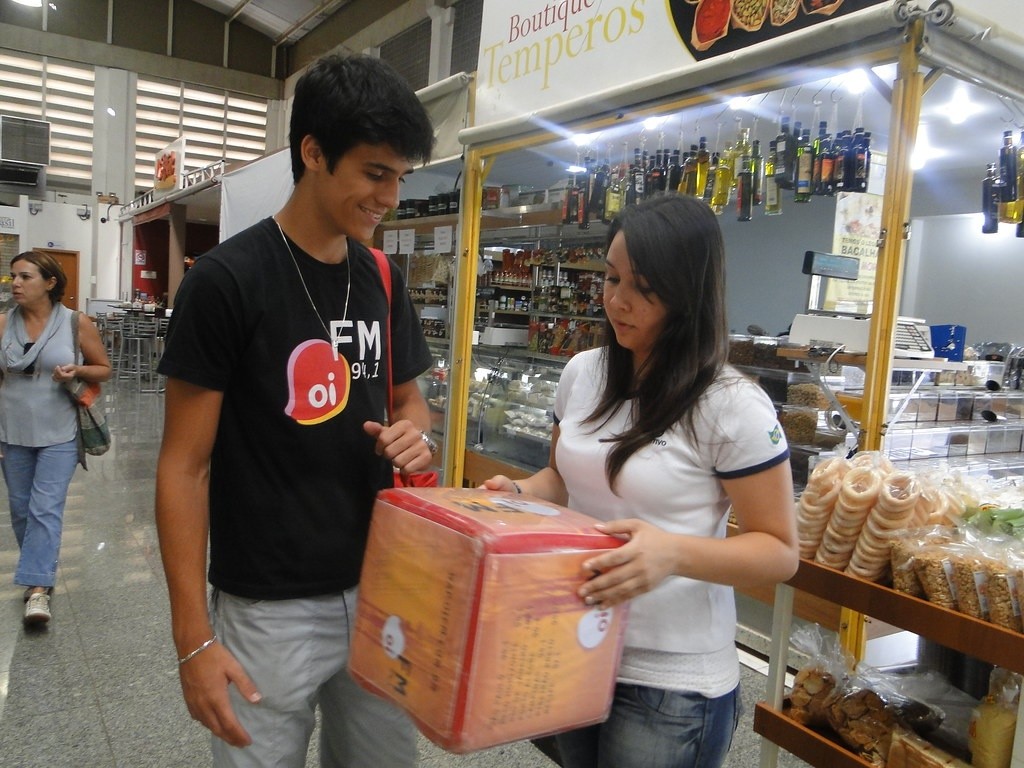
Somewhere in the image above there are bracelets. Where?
[178,635,217,663]
[511,482,522,493]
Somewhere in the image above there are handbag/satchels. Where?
[63,310,111,456]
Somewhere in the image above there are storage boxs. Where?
[348,488,631,756]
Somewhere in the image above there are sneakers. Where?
[24,592,51,624]
[22,586,37,602]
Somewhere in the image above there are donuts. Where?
[796,452,949,583]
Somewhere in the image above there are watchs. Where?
[417,429,438,456]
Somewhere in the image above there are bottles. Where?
[559,94,871,230]
[476,266,614,354]
[981,123,1023,238]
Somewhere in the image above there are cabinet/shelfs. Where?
[727,333,1024,501]
[480,270,529,348]
[752,557,1024,768]
[416,336,574,473]
[529,257,606,357]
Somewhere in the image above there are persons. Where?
[153,57,435,766]
[0,251,112,623]
[483,194,800,767]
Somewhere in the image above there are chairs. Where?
[96,302,171,394]
